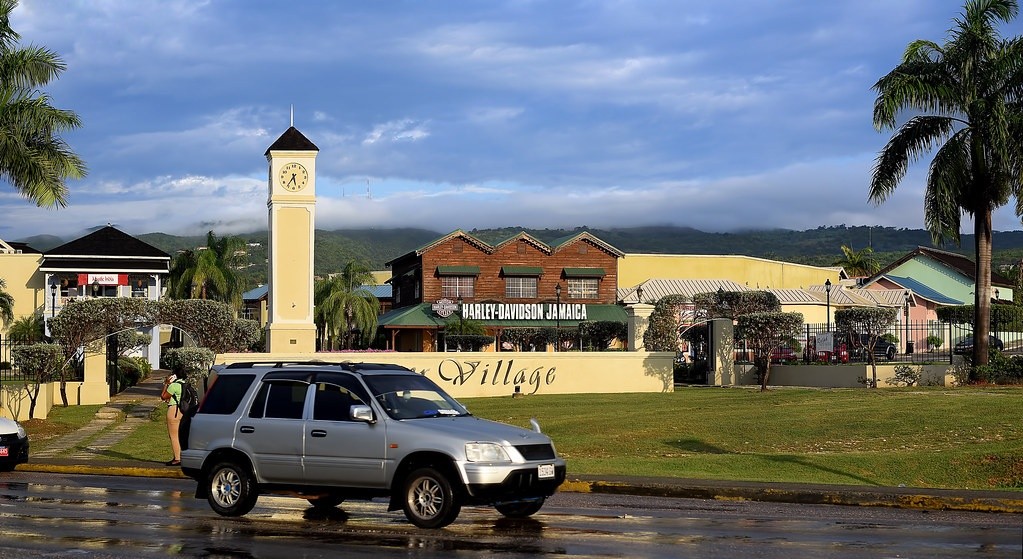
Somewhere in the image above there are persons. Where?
[161,366,187,466]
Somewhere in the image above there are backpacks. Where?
[172,381,198,417]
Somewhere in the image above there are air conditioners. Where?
[10,249,22,254]
[0,249,8,254]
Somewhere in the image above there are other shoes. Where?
[166,460,181,466]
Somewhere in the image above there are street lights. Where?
[457,294,464,346]
[903,290,911,357]
[993,286,1000,351]
[553,282,562,354]
[92,278,100,299]
[636,285,644,305]
[51,282,58,321]
[824,279,833,367]
[717,286,726,316]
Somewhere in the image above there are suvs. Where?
[843,333,897,359]
[179,360,569,531]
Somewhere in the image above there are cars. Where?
[0,415,30,473]
[803,336,849,364]
[767,341,798,363]
[674,347,687,377]
[954,333,1004,356]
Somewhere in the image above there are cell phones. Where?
[168,374,177,383]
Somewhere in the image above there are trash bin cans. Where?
[907,343,914,354]
[161,342,183,367]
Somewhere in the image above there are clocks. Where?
[279,162,308,192]
